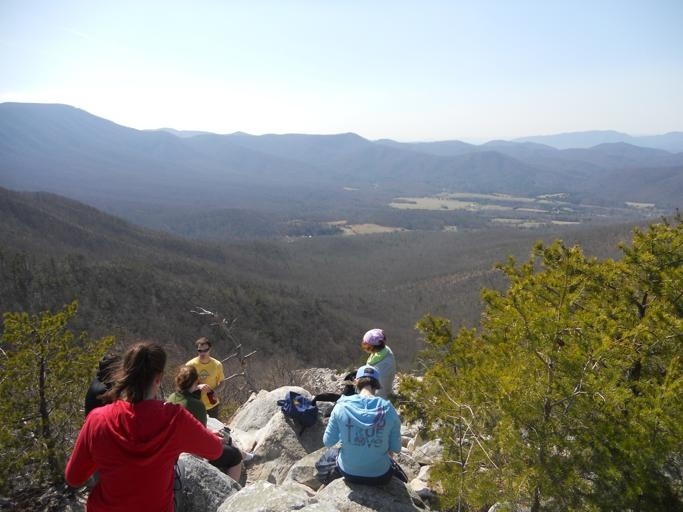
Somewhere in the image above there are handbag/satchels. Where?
[277,390,319,425]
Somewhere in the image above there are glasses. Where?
[197,347,210,353]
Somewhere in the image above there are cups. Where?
[208,390,218,405]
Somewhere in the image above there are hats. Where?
[362,328,385,346]
[355,365,380,382]
[98,352,121,369]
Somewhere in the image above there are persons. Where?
[61,341,223,511]
[358,327,397,395]
[309,363,408,485]
[165,362,206,431]
[176,335,223,420]
[201,429,244,489]
[82,351,122,420]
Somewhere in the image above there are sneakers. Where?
[386,456,407,484]
